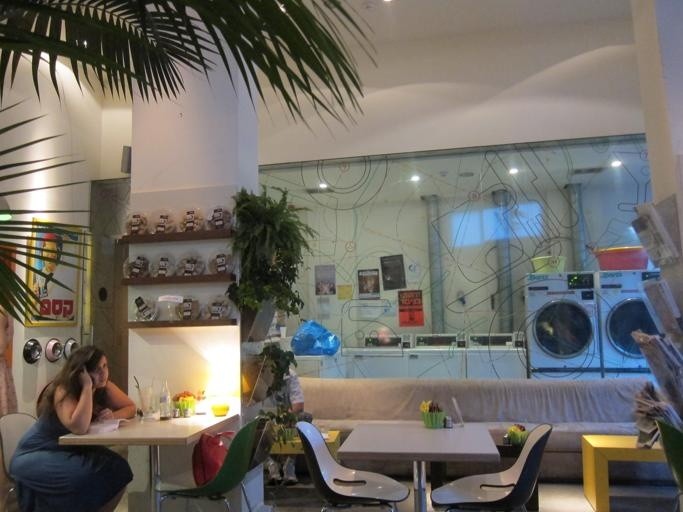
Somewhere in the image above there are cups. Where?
[279,326,286,338]
[171,408,179,418]
[182,408,190,418]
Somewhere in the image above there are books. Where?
[616,193,683,450]
[87,417,132,434]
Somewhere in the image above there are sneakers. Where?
[268,461,297,486]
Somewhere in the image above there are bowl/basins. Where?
[530,256,567,274]
[209,403,228,416]
[592,247,649,271]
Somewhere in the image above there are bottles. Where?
[159,379,170,421]
[122,205,236,318]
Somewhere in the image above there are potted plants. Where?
[233,184,320,342]
[241,347,298,448]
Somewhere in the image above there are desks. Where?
[271,430,341,466]
[582,435,669,512]
[57,411,239,512]
[337,425,500,512]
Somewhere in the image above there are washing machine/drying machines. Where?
[595,268,664,377]
[525,270,601,380]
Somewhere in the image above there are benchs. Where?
[295,379,676,484]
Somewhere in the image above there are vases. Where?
[177,402,195,416]
[423,413,444,427]
[284,429,296,439]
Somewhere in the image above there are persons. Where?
[9,345,137,510]
[257,358,304,487]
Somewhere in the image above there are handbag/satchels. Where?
[192,432,232,486]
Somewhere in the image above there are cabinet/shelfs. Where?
[120,229,237,328]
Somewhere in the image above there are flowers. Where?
[418,399,443,412]
[173,390,196,402]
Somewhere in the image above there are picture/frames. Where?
[26,218,83,326]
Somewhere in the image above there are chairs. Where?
[0,414,41,512]
[295,421,410,512]
[431,424,553,512]
[156,416,263,512]
